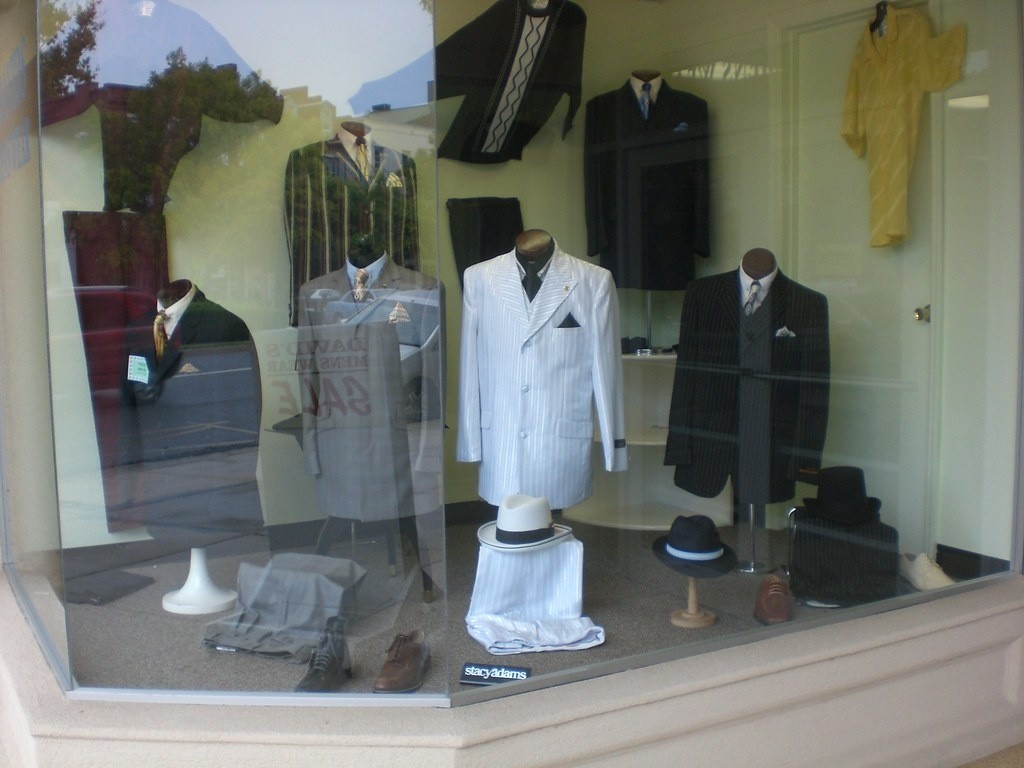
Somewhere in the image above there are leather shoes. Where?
[754,574,791,625]
[901,552,958,591]
[372,630,432,694]
[294,615,353,692]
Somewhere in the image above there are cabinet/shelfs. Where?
[560,352,735,532]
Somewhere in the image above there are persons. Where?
[118,278,266,543]
[664,247,830,503]
[297,232,448,521]
[582,65,712,292]
[460,227,636,514]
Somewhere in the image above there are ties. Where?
[516,245,554,303]
[353,269,369,301]
[641,83,653,120]
[153,311,168,362]
[743,281,761,316]
[354,135,372,182]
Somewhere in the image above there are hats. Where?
[652,514,737,578]
[802,465,882,526]
[475,493,573,553]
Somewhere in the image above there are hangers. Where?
[869,0,887,34]
